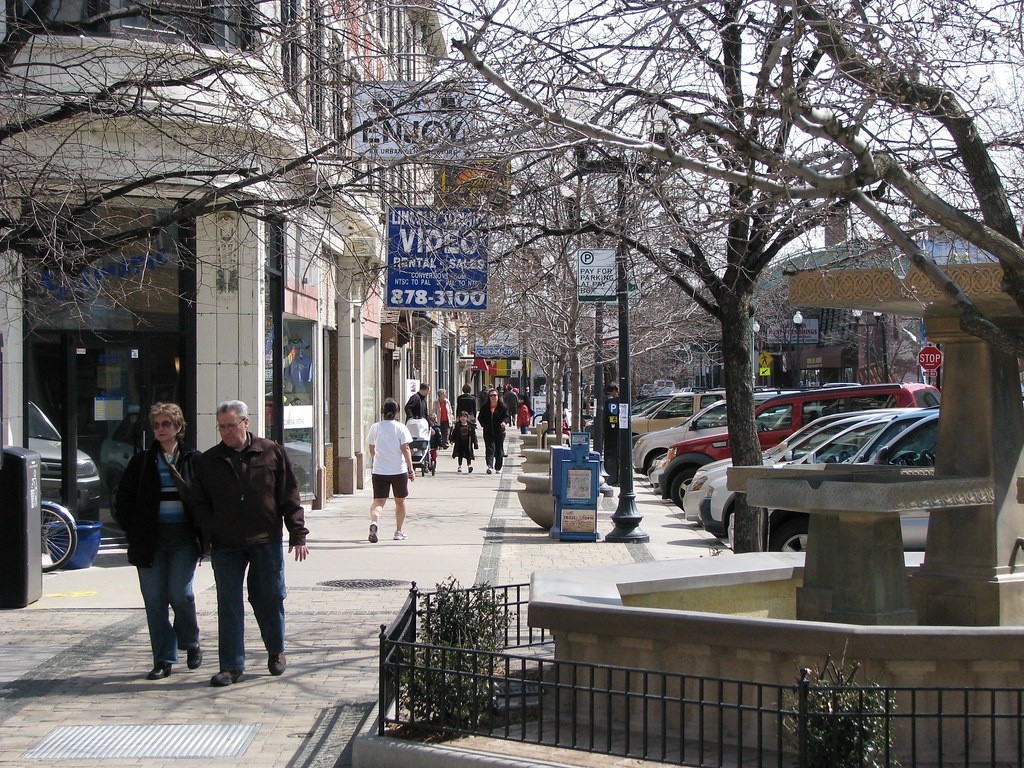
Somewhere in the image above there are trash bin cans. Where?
[0,445,43,609]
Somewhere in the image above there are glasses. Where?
[152,420,174,430]
[215,419,246,433]
[489,394,498,397]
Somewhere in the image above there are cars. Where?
[98,406,150,525]
[631,380,946,556]
[27,399,102,552]
[280,436,314,492]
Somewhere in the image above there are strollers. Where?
[404,417,438,479]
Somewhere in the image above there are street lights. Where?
[848,309,882,385]
[793,311,803,388]
[582,158,652,544]
[750,315,762,389]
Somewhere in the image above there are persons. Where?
[428,413,443,460]
[477,389,509,474]
[114,402,202,680]
[450,411,479,473]
[610,386,619,398]
[368,399,416,543]
[404,383,435,473]
[433,389,453,450]
[563,415,570,447]
[456,384,476,421]
[477,384,530,434]
[192,400,309,686]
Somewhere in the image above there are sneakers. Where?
[210,668,246,685]
[267,649,287,676]
[368,521,379,543]
[393,531,408,540]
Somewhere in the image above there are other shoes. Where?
[487,467,493,474]
[496,470,500,473]
[509,423,511,427]
[513,421,515,426]
[458,467,462,472]
[468,466,473,473]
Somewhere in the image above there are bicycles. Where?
[37,499,80,575]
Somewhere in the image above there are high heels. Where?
[187,640,202,669]
[147,662,172,680]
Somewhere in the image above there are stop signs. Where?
[917,346,944,370]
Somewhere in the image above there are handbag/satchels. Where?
[529,408,535,416]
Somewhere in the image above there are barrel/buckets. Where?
[43,519,103,570]
